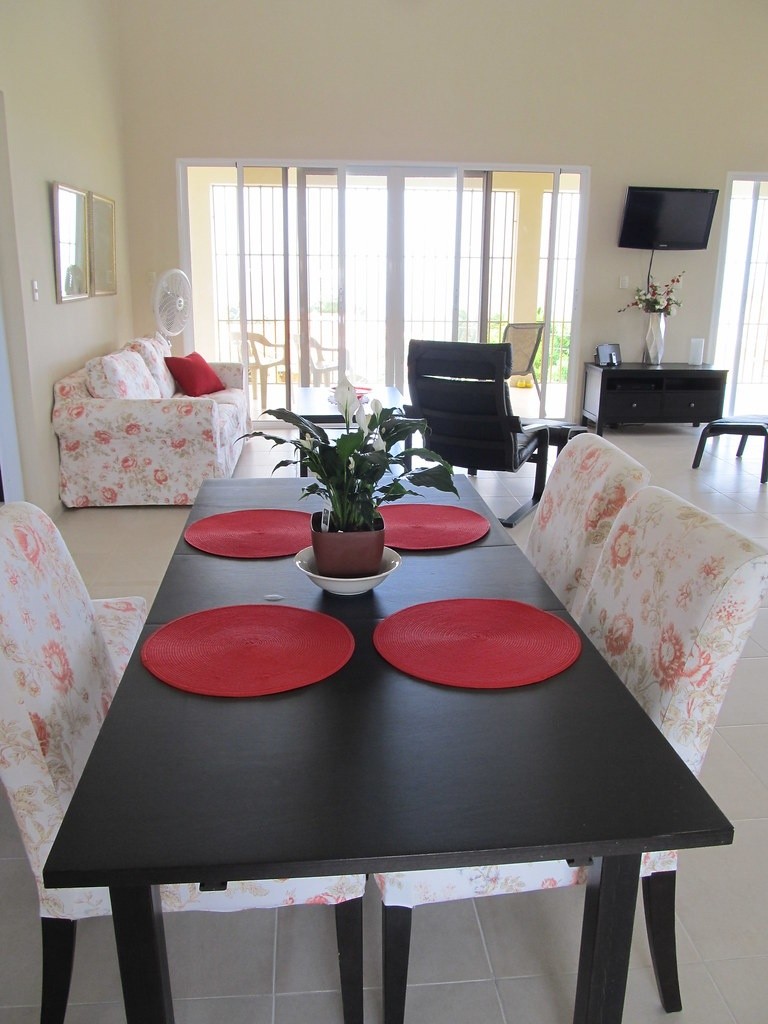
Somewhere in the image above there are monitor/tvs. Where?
[618,186,720,250]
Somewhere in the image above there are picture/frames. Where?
[88,189,117,297]
[51,180,90,304]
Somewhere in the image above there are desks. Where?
[42,471,733,1024]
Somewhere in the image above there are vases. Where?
[294,510,406,596]
[645,312,666,365]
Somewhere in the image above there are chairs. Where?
[525,432,652,623]
[229,331,296,411]
[0,502,370,1024]
[373,480,768,1024]
[501,322,547,417]
[91,591,149,675]
[691,414,768,484]
[303,331,351,388]
[400,337,550,530]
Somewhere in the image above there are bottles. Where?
[689,338,705,365]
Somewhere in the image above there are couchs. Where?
[51,332,250,509]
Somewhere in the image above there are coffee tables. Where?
[293,385,414,477]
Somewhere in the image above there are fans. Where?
[150,267,194,338]
[63,265,84,295]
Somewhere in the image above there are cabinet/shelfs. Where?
[583,362,729,439]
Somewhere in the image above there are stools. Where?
[521,415,589,460]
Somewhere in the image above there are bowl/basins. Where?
[294,545,403,595]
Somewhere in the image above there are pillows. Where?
[164,350,226,397]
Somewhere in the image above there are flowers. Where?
[617,269,687,318]
[232,372,460,530]
